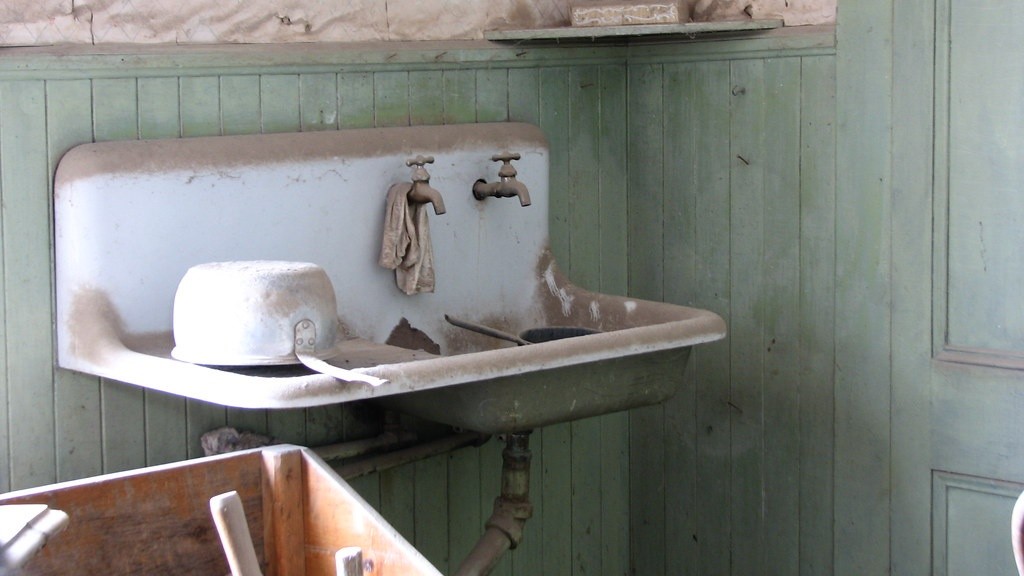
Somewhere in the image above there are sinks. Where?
[57,280,728,436]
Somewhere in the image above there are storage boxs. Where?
[0,443,440,576]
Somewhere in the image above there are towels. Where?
[376,181,436,296]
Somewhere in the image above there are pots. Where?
[171,261,390,387]
[445,312,604,346]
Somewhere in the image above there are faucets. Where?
[405,154,447,216]
[472,150,532,207]
